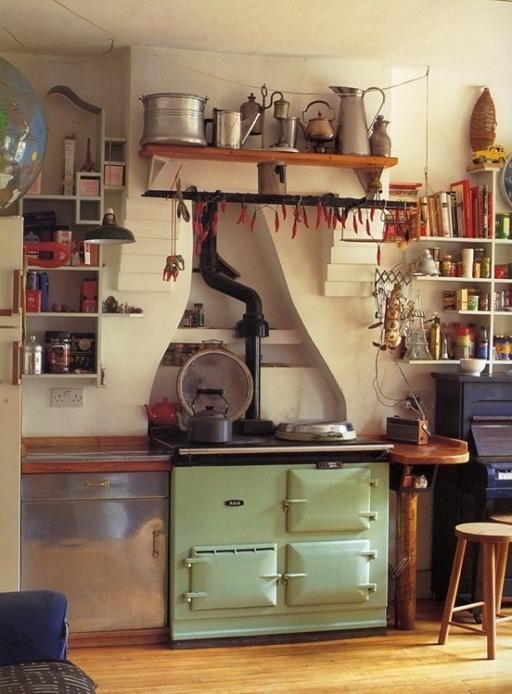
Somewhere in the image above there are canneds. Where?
[494,334,512,360]
[442,255,462,277]
[27,271,38,289]
[468,289,479,311]
[45,330,71,374]
[474,248,485,263]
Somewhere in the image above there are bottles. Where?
[194,302,204,326]
[494,334,512,361]
[467,86,496,152]
[414,249,440,274]
[441,255,452,276]
[184,308,195,328]
[483,256,491,277]
[21,225,98,373]
[426,317,488,360]
[240,91,261,134]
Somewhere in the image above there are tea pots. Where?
[144,396,183,427]
[175,387,234,446]
[328,84,385,155]
[204,106,260,149]
[370,115,391,156]
[298,98,337,153]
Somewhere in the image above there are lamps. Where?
[375,247,441,287]
[82,38,135,247]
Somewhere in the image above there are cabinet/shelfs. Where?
[19,83,145,390]
[409,164,512,377]
[21,470,169,652]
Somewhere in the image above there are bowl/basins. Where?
[458,357,486,377]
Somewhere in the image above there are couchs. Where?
[1,588,99,694]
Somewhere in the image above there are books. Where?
[421,179,495,240]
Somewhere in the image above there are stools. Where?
[491,510,509,614]
[435,521,510,661]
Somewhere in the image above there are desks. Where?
[358,433,473,630]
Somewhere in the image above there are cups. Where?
[467,290,511,311]
[451,247,480,277]
[257,158,287,195]
[279,116,299,147]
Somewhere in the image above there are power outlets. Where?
[50,386,83,408]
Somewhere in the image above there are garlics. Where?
[386,289,402,348]
